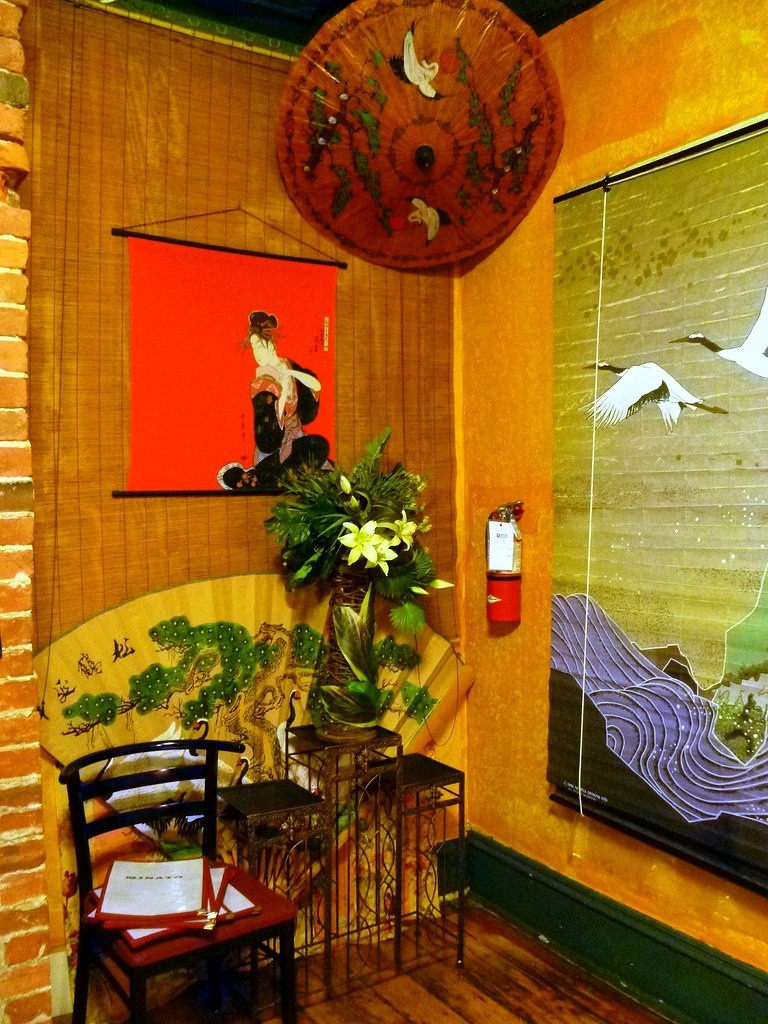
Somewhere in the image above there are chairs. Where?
[59,738,298,1024]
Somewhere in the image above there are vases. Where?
[306,585,382,743]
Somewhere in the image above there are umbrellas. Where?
[275,0,566,268]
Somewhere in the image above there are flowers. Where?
[261,434,454,599]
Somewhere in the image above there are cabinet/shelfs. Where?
[218,725,465,1010]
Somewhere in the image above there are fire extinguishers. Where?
[485,499,525,624]
